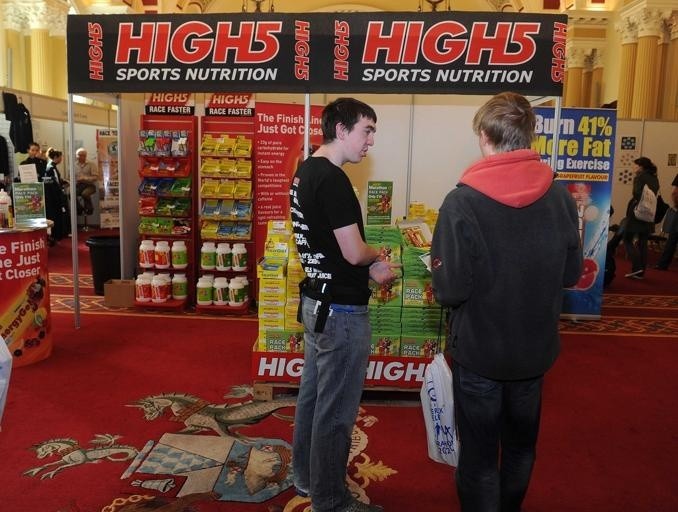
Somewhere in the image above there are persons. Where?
[0,135,98,241]
[624,157,660,280]
[289,97,401,512]
[432,93,583,511]
[652,173,678,270]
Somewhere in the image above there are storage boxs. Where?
[103,278,137,307]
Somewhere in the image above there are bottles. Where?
[133,272,190,304]
[138,239,189,270]
[200,241,251,272]
[194,274,249,307]
[0,188,13,229]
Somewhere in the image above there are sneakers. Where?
[624,267,644,281]
[335,489,380,512]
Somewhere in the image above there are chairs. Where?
[647,209,676,258]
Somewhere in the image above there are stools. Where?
[75,193,95,234]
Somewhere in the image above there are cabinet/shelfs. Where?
[134,112,198,315]
[199,115,254,314]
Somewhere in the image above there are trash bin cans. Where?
[86,235,121,296]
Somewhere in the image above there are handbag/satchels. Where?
[662,208,678,233]
[420,349,461,469]
[655,194,669,223]
[633,183,656,222]
